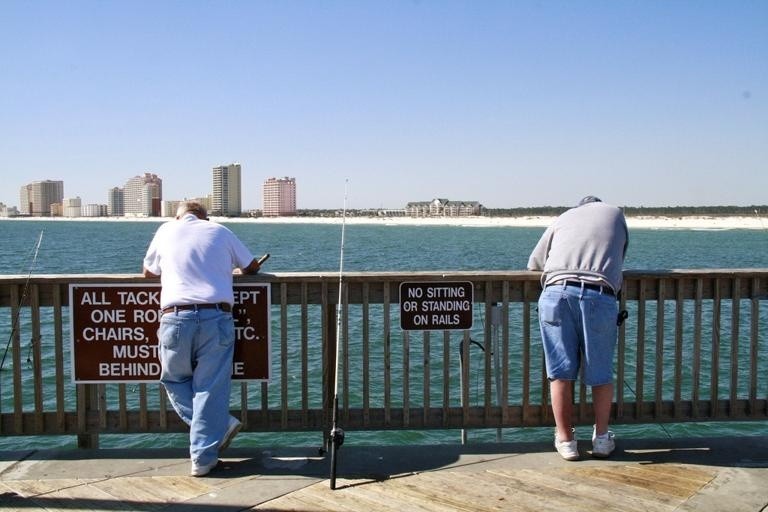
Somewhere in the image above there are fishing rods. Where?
[1,231,43,375]
[320,181,346,490]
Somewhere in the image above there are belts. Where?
[163,305,222,312]
[554,280,614,295]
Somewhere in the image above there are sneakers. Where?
[554,427,580,460]
[592,424,616,457]
[192,457,218,476]
[219,419,242,451]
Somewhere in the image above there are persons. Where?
[521,191,630,464]
[140,201,260,480]
